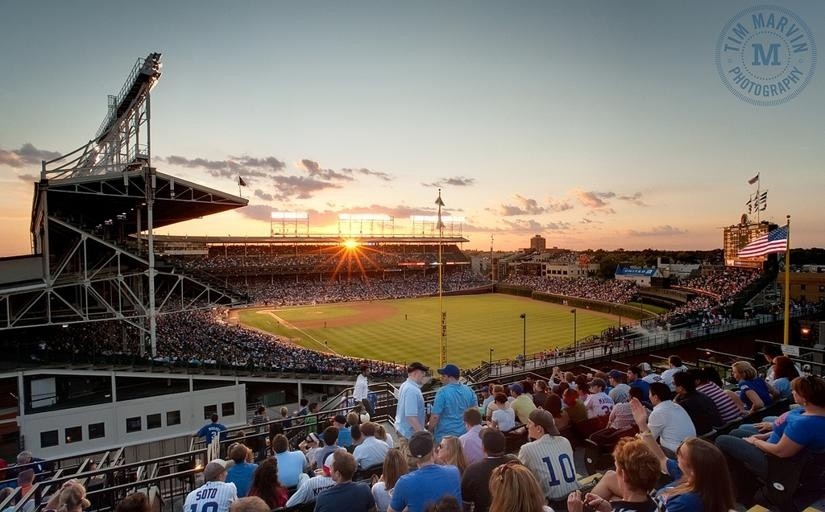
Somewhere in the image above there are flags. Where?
[748,175,759,185]
[738,224,789,258]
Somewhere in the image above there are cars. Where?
[781,263,825,274]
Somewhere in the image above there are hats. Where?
[508,384,522,393]
[479,428,506,451]
[206,459,234,480]
[438,365,459,378]
[408,363,429,373]
[638,363,652,374]
[409,430,433,458]
[529,408,560,435]
[587,371,622,385]
[331,415,346,424]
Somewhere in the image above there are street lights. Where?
[519,312,528,359]
[488,347,495,363]
[96,51,163,152]
[569,307,578,348]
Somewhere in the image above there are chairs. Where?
[270,382,825,512]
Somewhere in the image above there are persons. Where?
[539,348,559,367]
[17,286,294,370]
[659,297,757,338]
[393,361,825,512]
[679,267,763,302]
[157,244,486,304]
[773,299,817,318]
[352,367,375,418]
[505,271,642,306]
[1,450,103,512]
[117,492,149,512]
[600,326,630,354]
[290,348,407,374]
[184,399,393,512]
